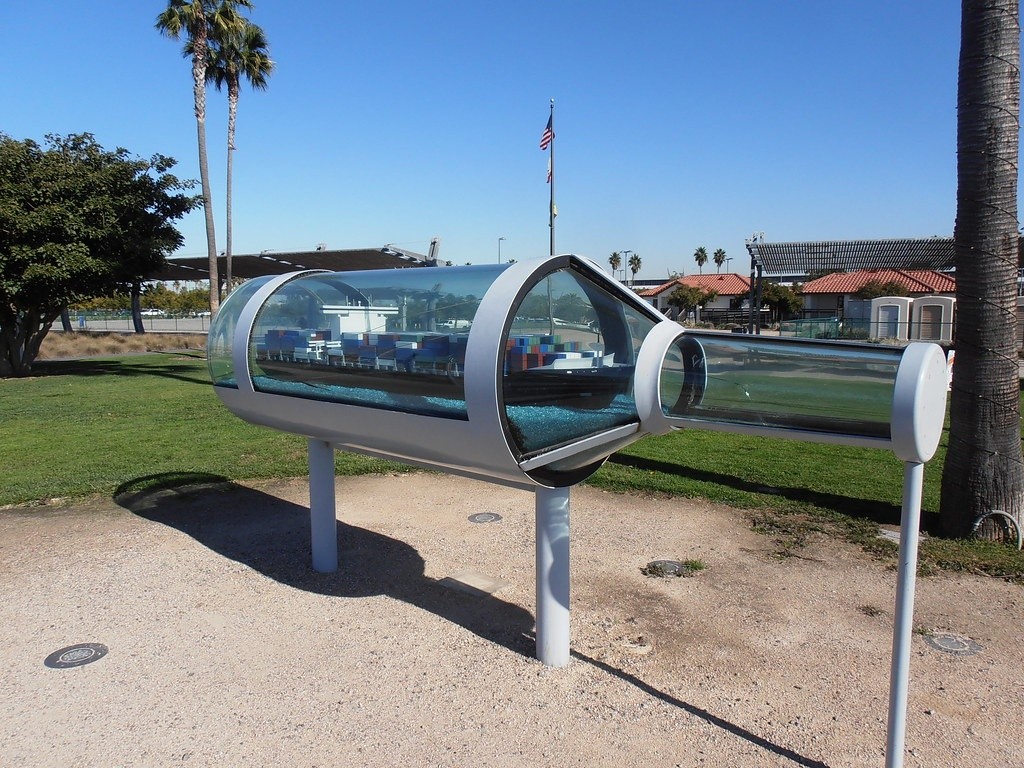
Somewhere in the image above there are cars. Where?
[189,310,211,318]
[140,308,165,316]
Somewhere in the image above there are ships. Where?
[253,303,638,410]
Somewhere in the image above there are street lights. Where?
[620,249,633,287]
[498,237,506,264]
[725,257,734,274]
[617,269,624,282]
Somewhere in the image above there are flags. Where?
[539,112,555,151]
